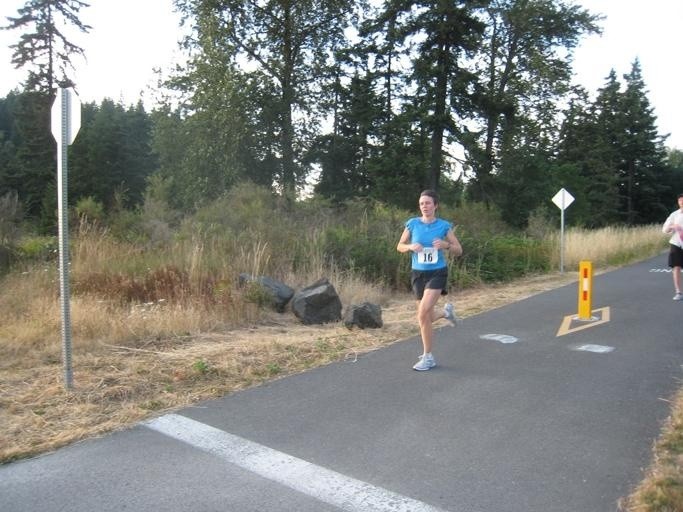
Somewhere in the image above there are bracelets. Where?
[446,240,451,250]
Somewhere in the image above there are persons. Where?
[397,190,462,371]
[662,194,683,300]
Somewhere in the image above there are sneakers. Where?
[413,354,436,371]
[673,293,683,300]
[444,304,457,327]
